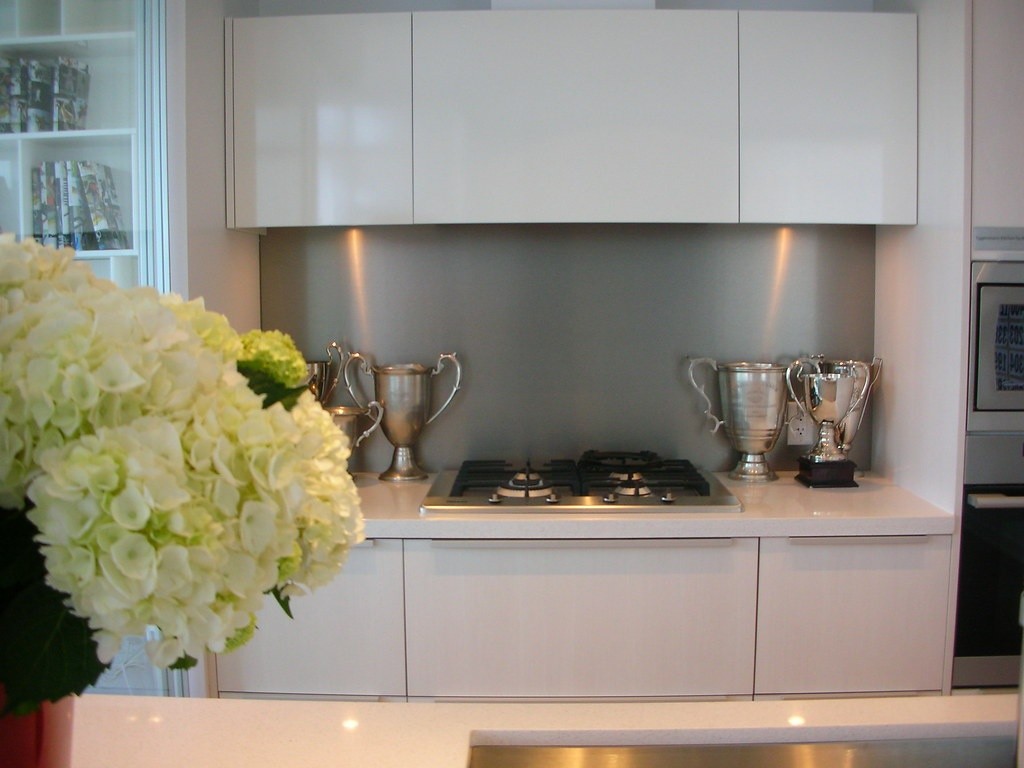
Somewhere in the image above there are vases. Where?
[0,684,75,768]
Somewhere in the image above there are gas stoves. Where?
[418,450,745,513]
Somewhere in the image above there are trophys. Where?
[324,401,384,453]
[308,343,345,409]
[689,358,805,483]
[784,354,882,489]
[345,353,462,480]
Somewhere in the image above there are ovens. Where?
[954,482,1024,691]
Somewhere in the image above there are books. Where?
[0,55,91,133]
[31,160,129,251]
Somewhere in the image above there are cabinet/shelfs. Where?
[0,0,156,289]
[208,538,407,705]
[753,535,953,701]
[401,538,758,699]
[224,10,919,236]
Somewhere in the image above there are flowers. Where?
[0,232,368,717]
